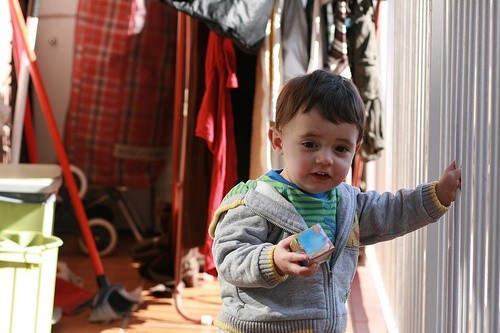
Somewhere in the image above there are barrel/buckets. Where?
[0,230,63,332]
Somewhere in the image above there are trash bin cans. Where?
[0,231,64,333]
[0,163,63,239]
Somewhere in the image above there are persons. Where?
[208,70,463,333]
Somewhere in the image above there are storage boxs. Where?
[1,163,63,238]
